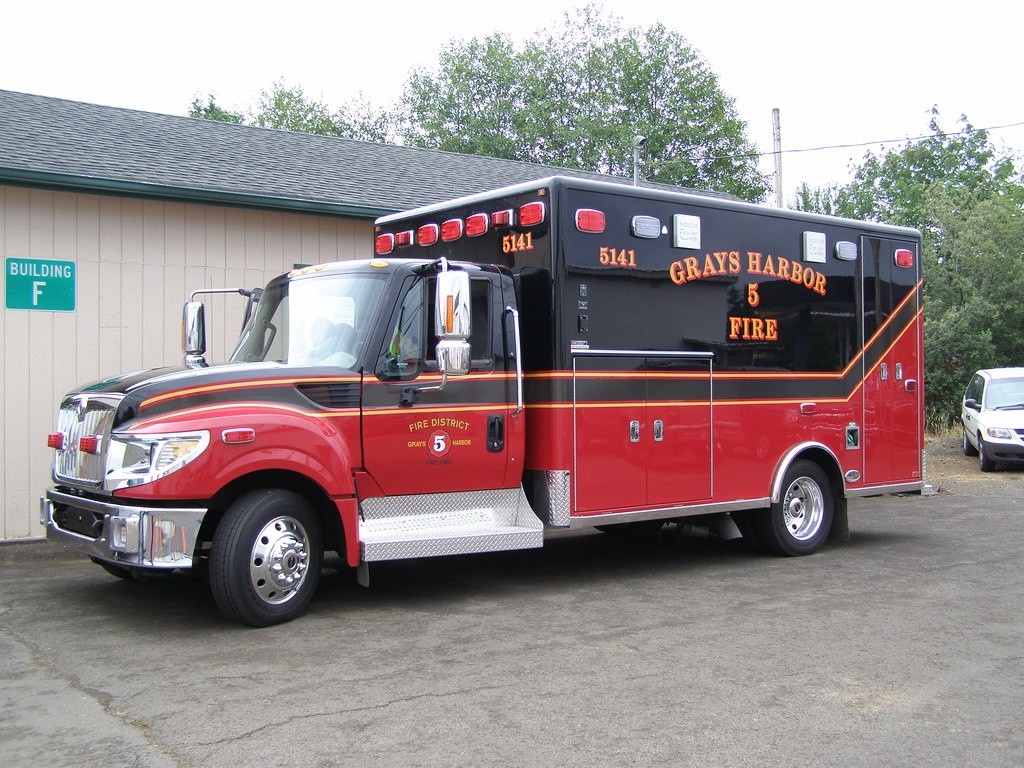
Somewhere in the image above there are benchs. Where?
[425,297,488,361]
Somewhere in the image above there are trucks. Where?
[41,176,936,628]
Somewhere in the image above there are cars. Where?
[961,367,1024,472]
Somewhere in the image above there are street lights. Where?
[633,135,646,187]
